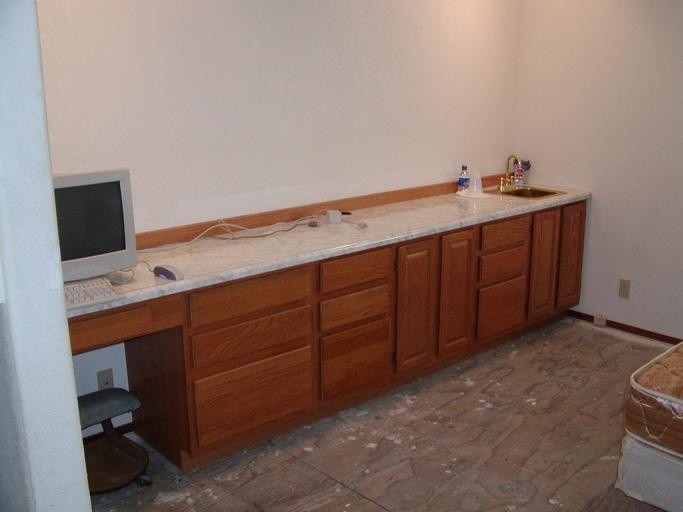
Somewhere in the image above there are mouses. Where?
[153,264,184,282]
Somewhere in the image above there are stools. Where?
[76,387,153,495]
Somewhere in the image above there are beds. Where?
[615,342,683,512]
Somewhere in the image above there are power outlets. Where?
[618,277,630,300]
[96,368,114,391]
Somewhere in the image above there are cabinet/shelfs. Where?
[129,196,588,475]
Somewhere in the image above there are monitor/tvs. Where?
[52,167,138,283]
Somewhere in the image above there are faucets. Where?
[505,154,526,187]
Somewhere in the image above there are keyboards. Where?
[63,276,122,310]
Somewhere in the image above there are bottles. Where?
[458,165,469,196]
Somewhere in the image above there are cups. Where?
[469,174,483,197]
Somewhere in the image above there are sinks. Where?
[483,187,568,200]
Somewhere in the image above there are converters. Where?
[326,209,342,224]
